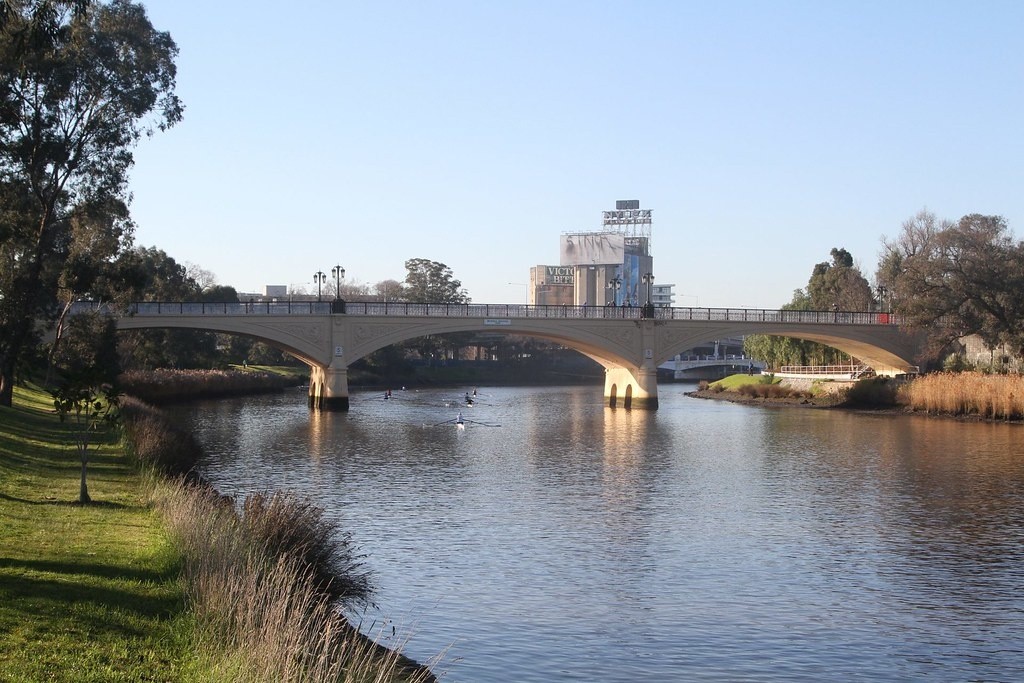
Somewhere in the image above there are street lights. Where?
[609,278,621,307]
[642,270,654,304]
[330,262,346,298]
[876,285,887,313]
[313,270,327,302]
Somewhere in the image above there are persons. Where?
[384,388,391,398]
[464,388,477,404]
[747,360,754,376]
[456,411,464,425]
[249,296,255,314]
